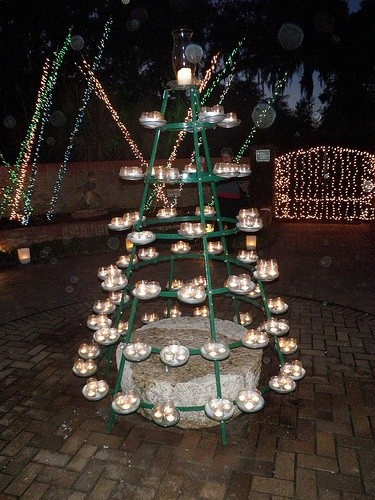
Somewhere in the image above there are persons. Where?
[204,140,277,262]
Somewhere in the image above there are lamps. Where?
[17,247,32,264]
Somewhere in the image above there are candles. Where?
[74,67,304,429]
